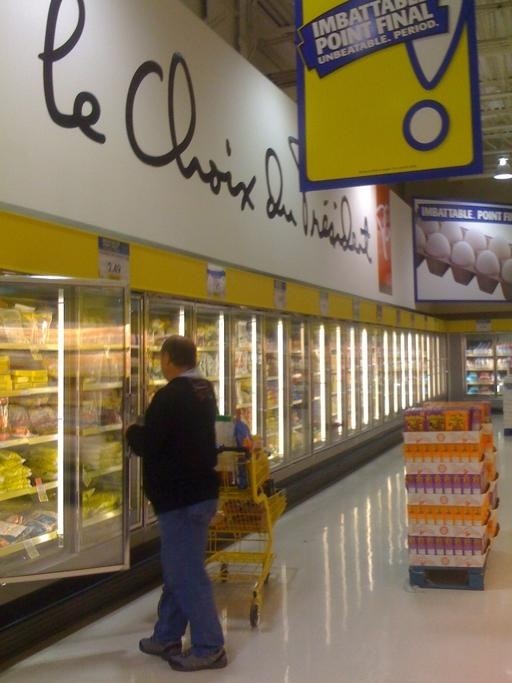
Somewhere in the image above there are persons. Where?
[123,333,229,672]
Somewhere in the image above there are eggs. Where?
[417,217,512,284]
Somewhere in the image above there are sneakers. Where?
[169,647,226,672]
[140,635,183,659]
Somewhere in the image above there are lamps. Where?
[493,155,512,180]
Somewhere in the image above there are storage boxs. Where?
[402,399,499,567]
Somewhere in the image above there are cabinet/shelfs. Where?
[465,355,512,395]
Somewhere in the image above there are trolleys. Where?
[200,445,288,627]
